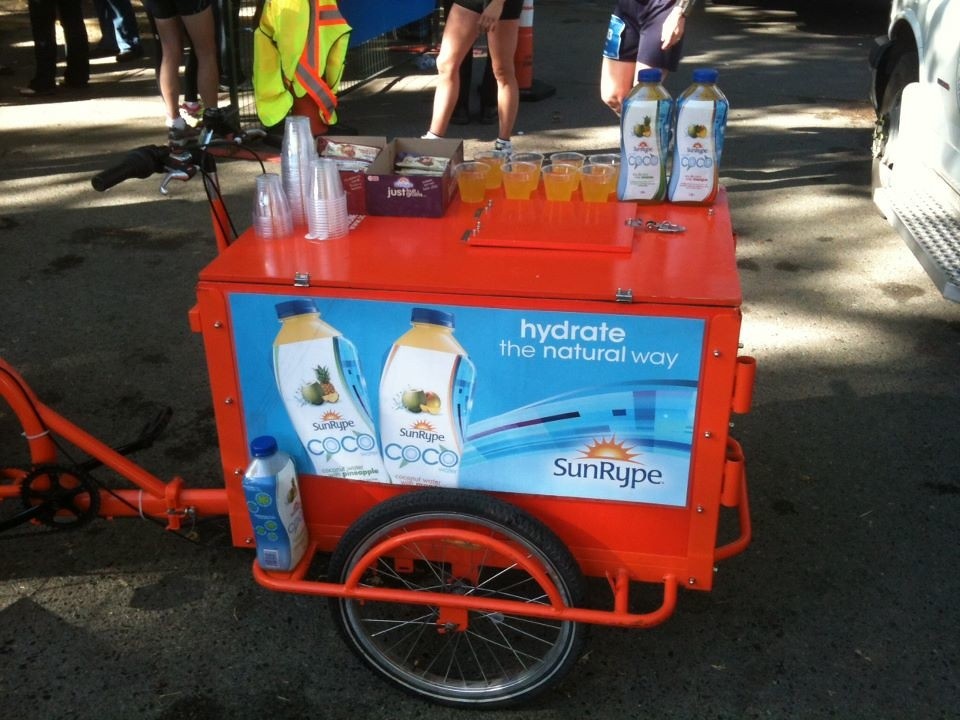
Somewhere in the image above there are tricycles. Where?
[2,108,755,705]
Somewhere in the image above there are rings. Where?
[677,38,680,40]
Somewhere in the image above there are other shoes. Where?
[492,141,512,156]
[89,47,120,58]
[56,81,88,91]
[19,78,56,96]
[116,50,142,62]
[181,103,201,116]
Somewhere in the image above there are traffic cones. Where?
[487,0,556,102]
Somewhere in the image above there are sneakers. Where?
[167,123,201,147]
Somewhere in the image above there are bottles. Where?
[615,67,674,202]
[273,299,391,483]
[667,69,729,204]
[377,308,477,487]
[393,50,435,70]
[240,436,310,572]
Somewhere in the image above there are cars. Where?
[858,1,960,312]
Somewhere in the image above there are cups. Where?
[577,164,617,202]
[540,164,579,201]
[500,162,538,200]
[509,151,544,189]
[472,150,508,188]
[550,152,586,191]
[455,162,491,203]
[304,158,350,239]
[251,173,294,239]
[588,154,620,193]
[280,115,320,225]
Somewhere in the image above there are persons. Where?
[601,0,695,118]
[421,0,523,162]
[20,0,236,145]
